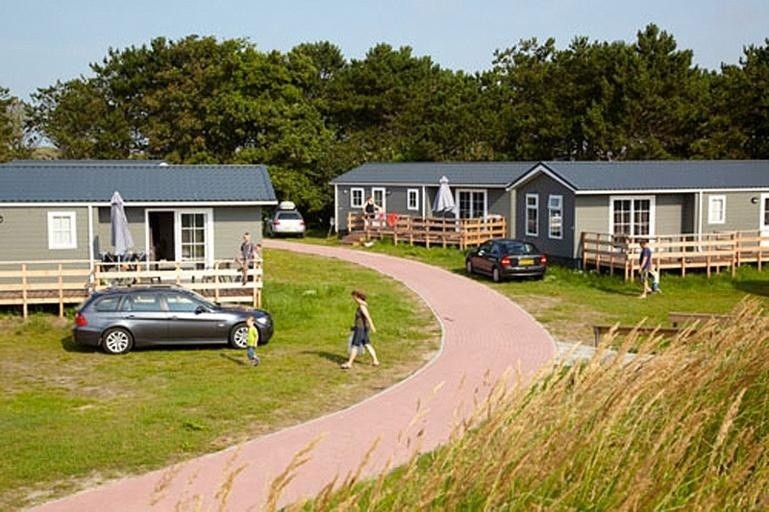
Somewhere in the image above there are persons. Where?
[363,196,375,233]
[649,265,666,295]
[341,290,381,369]
[235,232,253,285]
[254,243,263,281]
[244,314,262,367]
[373,202,386,230]
[636,240,652,298]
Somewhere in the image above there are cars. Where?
[74,283,275,354]
[466,239,547,283]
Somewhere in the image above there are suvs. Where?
[264,200,306,238]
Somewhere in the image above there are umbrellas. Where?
[110,191,134,272]
[432,176,457,218]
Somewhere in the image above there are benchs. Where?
[668,311,734,327]
[591,326,715,350]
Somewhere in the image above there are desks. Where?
[102,251,152,283]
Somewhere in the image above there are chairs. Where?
[200,257,233,293]
